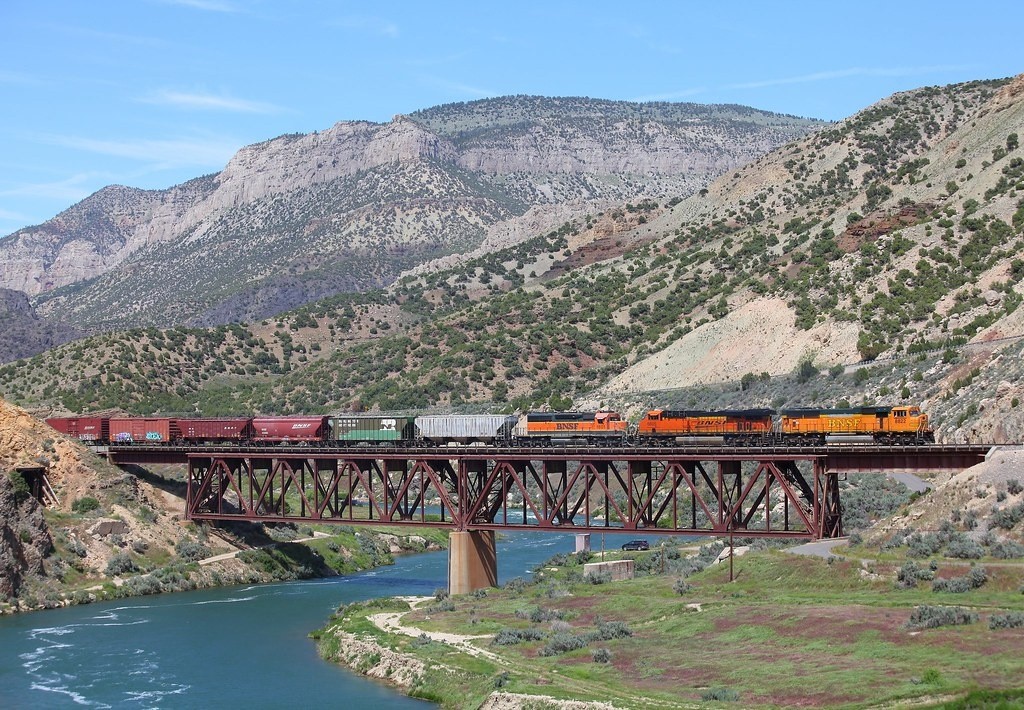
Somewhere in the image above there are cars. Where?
[339,499,362,506]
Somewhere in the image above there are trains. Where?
[46,405,936,448]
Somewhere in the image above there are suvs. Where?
[622,540,650,551]
